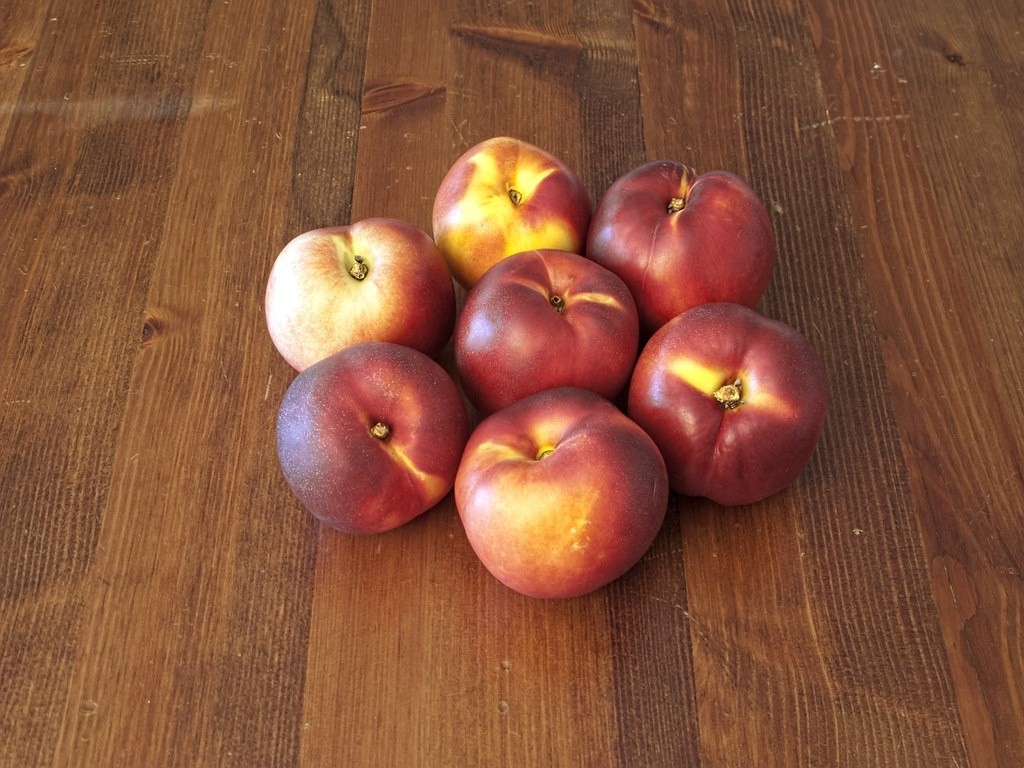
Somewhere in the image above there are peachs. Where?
[264,137,830,603]
